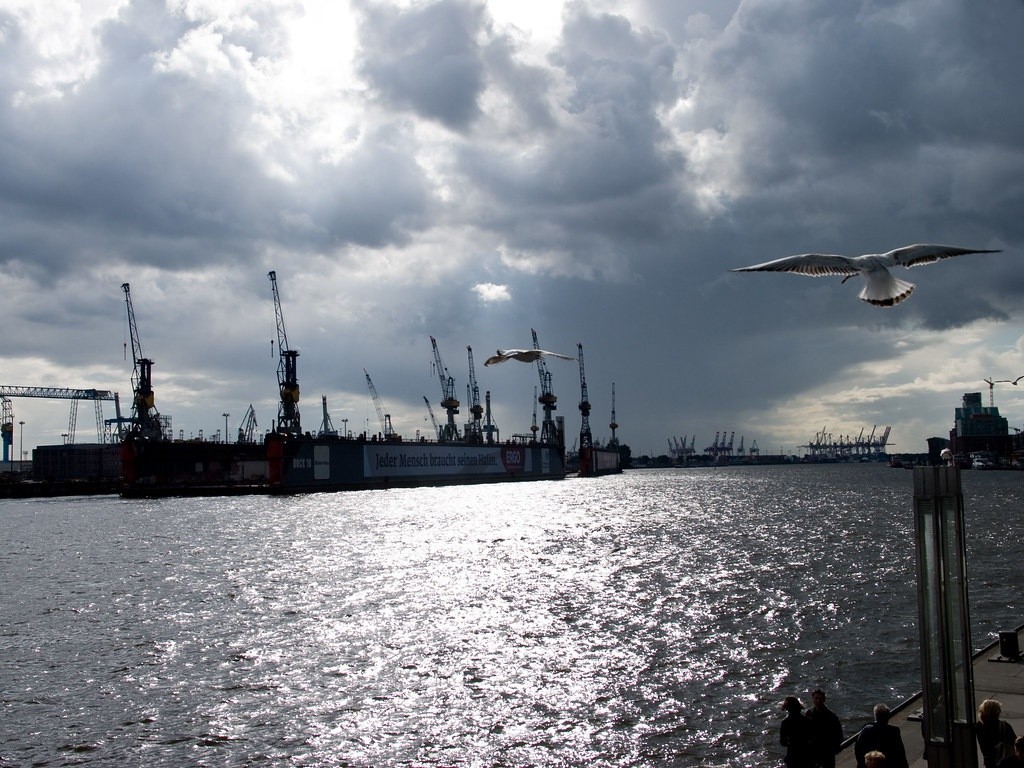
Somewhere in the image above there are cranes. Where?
[264,269,312,491]
[316,327,619,478]
[103,282,170,498]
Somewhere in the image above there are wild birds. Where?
[725,243,1007,311]
[993,375,1024,386]
[482,347,577,369]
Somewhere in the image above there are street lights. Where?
[18,421,25,473]
[222,411,231,444]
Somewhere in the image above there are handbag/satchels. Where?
[993,721,1015,766]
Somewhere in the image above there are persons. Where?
[779,688,844,768]
[854,703,909,768]
[976,698,1024,768]
